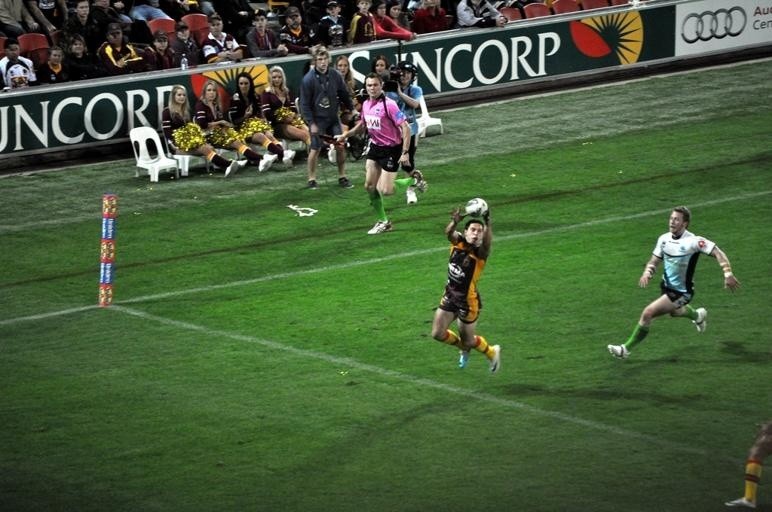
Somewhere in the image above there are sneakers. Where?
[307,179,320,190]
[692,307,707,333]
[409,170,428,195]
[723,498,758,509]
[406,188,419,205]
[257,153,279,173]
[367,218,393,235]
[224,157,248,178]
[338,177,354,190]
[282,149,297,169]
[487,344,501,372]
[607,343,632,362]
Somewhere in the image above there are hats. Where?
[251,8,266,19]
[326,0,340,8]
[106,21,124,35]
[208,13,223,22]
[174,20,190,31]
[285,7,301,18]
[151,28,170,40]
[399,60,419,74]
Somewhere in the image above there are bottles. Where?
[179,54,189,71]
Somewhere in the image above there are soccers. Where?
[465,198,489,217]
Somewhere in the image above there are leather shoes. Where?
[458,350,470,368]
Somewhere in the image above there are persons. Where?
[1,1,243,91]
[722,424,771,511]
[456,0,508,28]
[431,198,502,373]
[260,64,338,166]
[385,60,423,205]
[247,1,441,56]
[297,45,363,192]
[373,53,390,77]
[333,72,430,238]
[605,205,742,360]
[335,56,365,143]
[194,80,280,175]
[160,83,249,178]
[228,73,298,170]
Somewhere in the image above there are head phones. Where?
[398,60,418,82]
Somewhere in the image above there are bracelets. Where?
[641,270,653,280]
[723,271,733,278]
[401,150,410,156]
